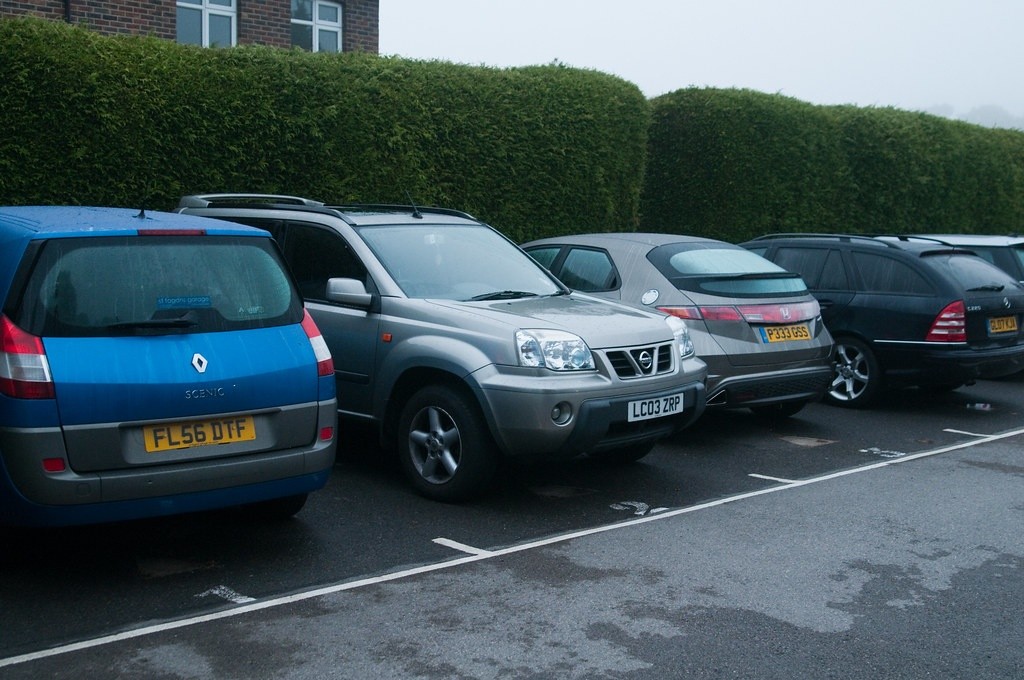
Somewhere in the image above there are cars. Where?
[0,205,338,570]
[516,232,838,433]
[874,232,1024,290]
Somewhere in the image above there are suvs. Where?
[736,232,1024,409]
[146,192,708,503]
